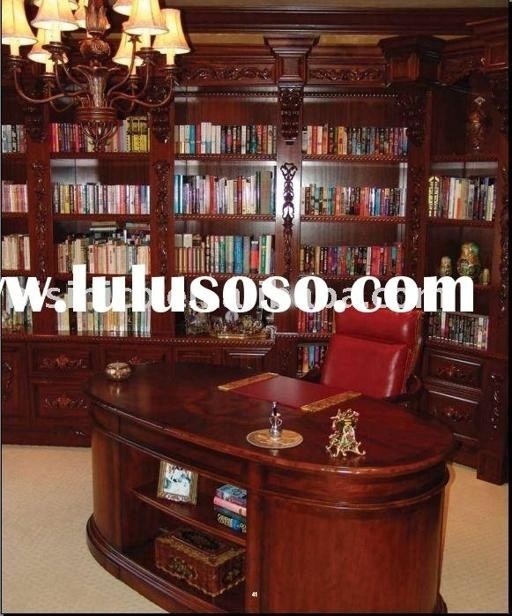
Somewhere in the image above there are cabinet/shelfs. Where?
[1,0,512,484]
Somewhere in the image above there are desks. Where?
[83,361,453,614]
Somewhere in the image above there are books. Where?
[427,307,489,352]
[0,116,408,343]
[213,483,247,534]
[295,341,328,380]
[427,172,496,221]
